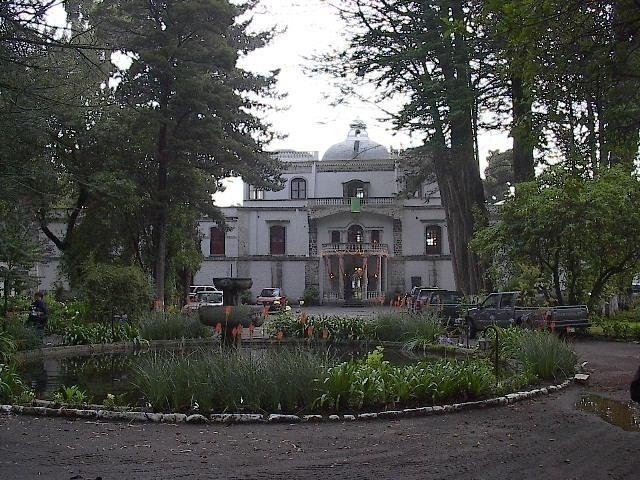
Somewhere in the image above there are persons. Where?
[28,292,48,330]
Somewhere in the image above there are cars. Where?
[183,284,224,310]
[394,285,476,326]
[256,287,288,312]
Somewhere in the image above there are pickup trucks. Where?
[458,291,592,339]
